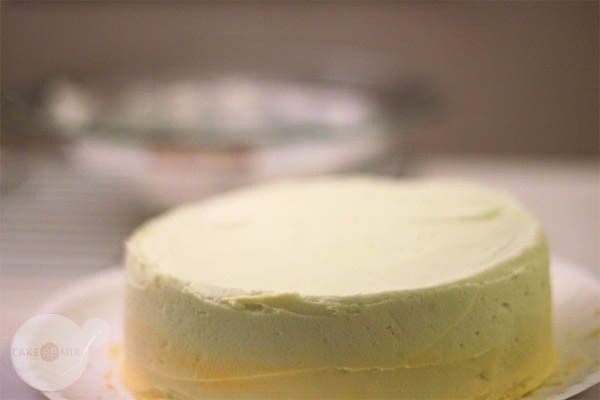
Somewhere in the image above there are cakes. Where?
[123,177,554,400]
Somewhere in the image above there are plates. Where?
[10,254,600,400]
